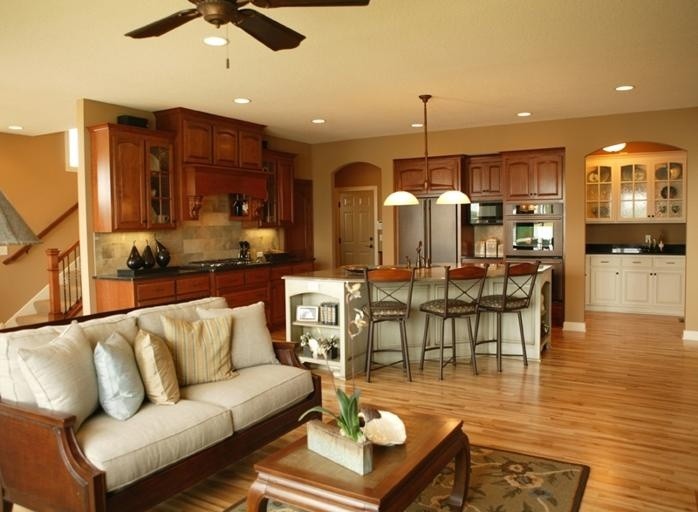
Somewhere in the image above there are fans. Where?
[122,0,370,52]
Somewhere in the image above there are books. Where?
[319,302,337,325]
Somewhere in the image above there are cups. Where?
[157,213,169,224]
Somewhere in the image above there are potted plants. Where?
[298,384,374,477]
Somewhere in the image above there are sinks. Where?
[395,265,431,270]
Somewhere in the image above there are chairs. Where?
[363,266,416,383]
[420,262,489,381]
[471,260,542,372]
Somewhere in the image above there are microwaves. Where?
[464,203,503,226]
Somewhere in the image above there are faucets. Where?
[413,240,422,268]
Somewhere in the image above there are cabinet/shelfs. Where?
[92,270,211,313]
[279,272,371,381]
[211,264,272,329]
[534,264,551,361]
[393,147,565,203]
[242,150,298,228]
[87,123,177,233]
[151,106,268,170]
[272,258,315,327]
[459,206,564,259]
[585,253,686,316]
[583,138,686,223]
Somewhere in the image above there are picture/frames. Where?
[296,304,320,323]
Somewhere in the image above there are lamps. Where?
[383,94,472,206]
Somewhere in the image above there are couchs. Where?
[0,298,322,512]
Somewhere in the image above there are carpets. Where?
[222,442,591,512]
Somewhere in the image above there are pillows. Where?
[17,300,281,433]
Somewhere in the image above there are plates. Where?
[358,408,407,448]
[632,167,646,181]
[660,184,678,198]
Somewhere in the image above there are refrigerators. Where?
[399,193,456,266]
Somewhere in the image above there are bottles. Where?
[265,198,273,222]
[233,192,249,216]
